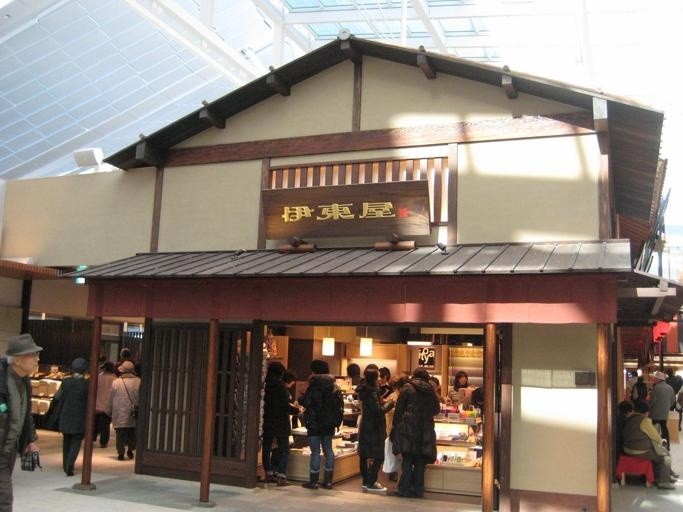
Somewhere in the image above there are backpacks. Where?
[313,378,345,431]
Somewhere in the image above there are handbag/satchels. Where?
[632,385,640,401]
[55,397,65,416]
[130,404,139,418]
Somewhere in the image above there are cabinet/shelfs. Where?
[22,365,68,434]
[286,389,360,486]
[424,413,483,497]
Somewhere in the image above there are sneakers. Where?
[670,475,677,482]
[64,443,134,476]
[263,471,275,483]
[657,481,676,490]
[362,480,369,489]
[277,474,292,487]
[366,481,388,492]
[672,470,680,476]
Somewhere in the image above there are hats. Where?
[119,348,132,358]
[4,333,44,357]
[117,361,137,374]
[309,359,329,374]
[647,370,665,381]
[412,366,429,380]
[635,401,649,413]
[71,357,89,373]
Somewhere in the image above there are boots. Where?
[302,470,319,489]
[319,468,333,489]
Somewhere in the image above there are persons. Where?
[261,358,484,497]
[45,356,90,477]
[92,347,141,461]
[619,368,682,491]
[0,332,44,512]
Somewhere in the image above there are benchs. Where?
[620,456,653,487]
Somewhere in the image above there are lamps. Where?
[359,325,373,357]
[322,326,335,357]
